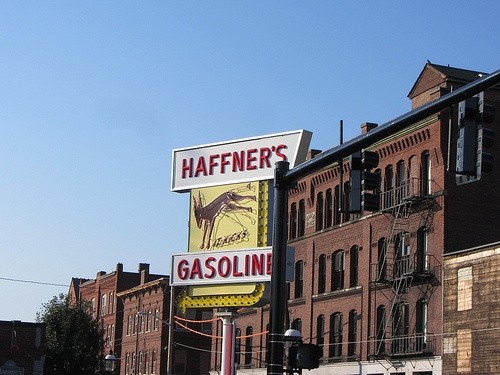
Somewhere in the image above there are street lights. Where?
[282,318,320,375]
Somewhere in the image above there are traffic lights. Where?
[455,91,484,187]
[347,150,381,214]
